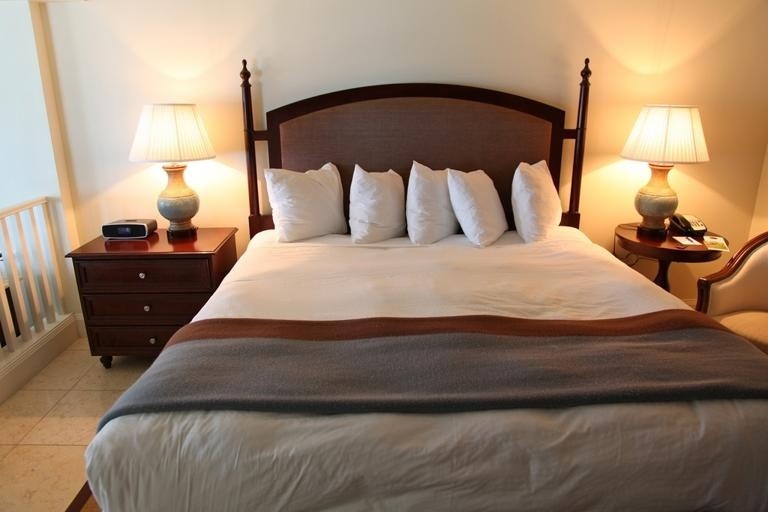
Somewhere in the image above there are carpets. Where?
[65,480,100,512]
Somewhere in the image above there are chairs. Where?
[696,232,768,358]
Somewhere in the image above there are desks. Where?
[615,221,728,295]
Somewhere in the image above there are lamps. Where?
[623,105,710,241]
[130,104,217,241]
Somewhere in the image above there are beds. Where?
[86,59,767,512]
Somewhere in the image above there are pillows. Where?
[509,159,563,248]
[347,163,406,245]
[447,163,509,248]
[405,162,460,247]
[264,162,348,243]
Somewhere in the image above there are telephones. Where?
[670,214,707,236]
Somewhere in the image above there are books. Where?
[672,235,730,251]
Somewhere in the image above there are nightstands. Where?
[64,227,240,369]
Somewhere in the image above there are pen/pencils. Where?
[687,237,693,242]
